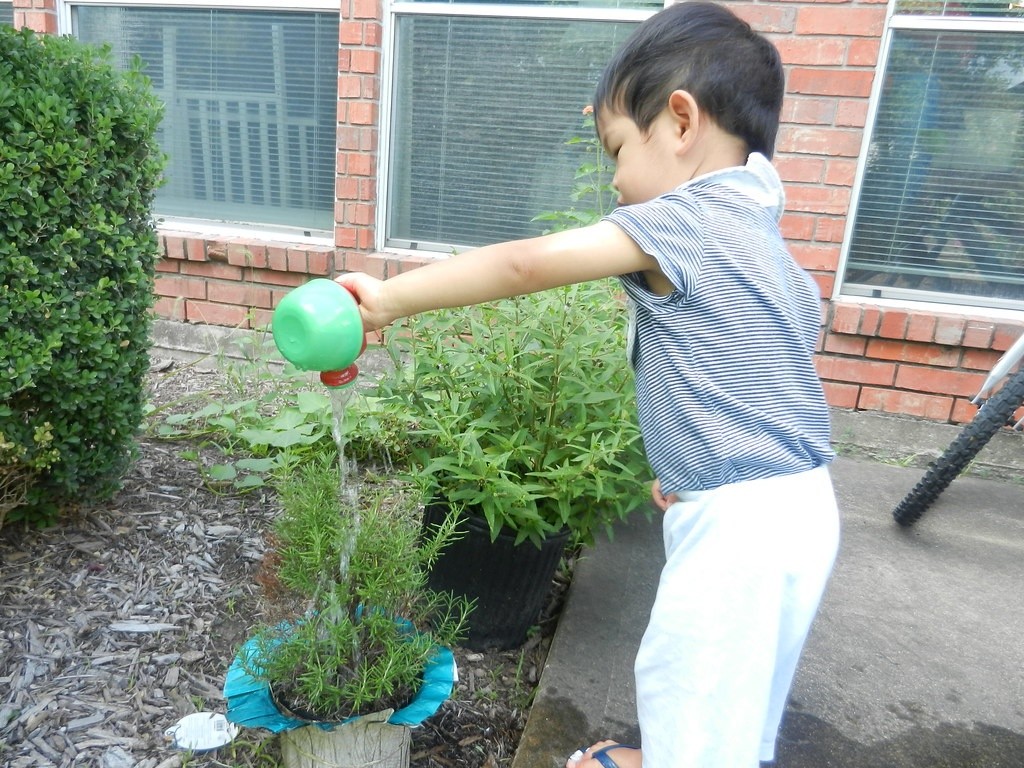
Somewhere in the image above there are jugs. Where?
[272,277,367,389]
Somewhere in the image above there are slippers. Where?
[566,742,637,768]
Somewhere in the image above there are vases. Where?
[415,480,572,656]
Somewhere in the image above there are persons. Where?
[336,1,841,768]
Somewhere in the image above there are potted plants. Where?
[222,453,459,768]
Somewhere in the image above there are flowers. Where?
[365,104,657,557]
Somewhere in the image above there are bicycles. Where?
[882,187,1024,299]
[890,335,1024,529]
